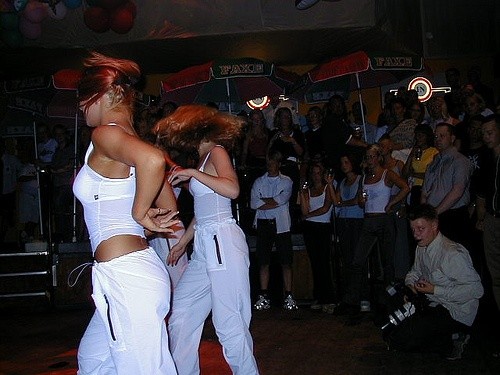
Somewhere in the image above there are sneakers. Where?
[445,332,470,360]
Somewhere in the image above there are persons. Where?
[1,82,500,375]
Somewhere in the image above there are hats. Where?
[51,69,82,92]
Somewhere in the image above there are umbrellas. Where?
[0,49,162,246]
[160,49,299,169]
[298,49,423,143]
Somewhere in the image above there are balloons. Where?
[0,0,136,40]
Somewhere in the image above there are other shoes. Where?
[310,301,322,310]
[252,295,271,310]
[283,295,298,310]
[359,301,371,312]
